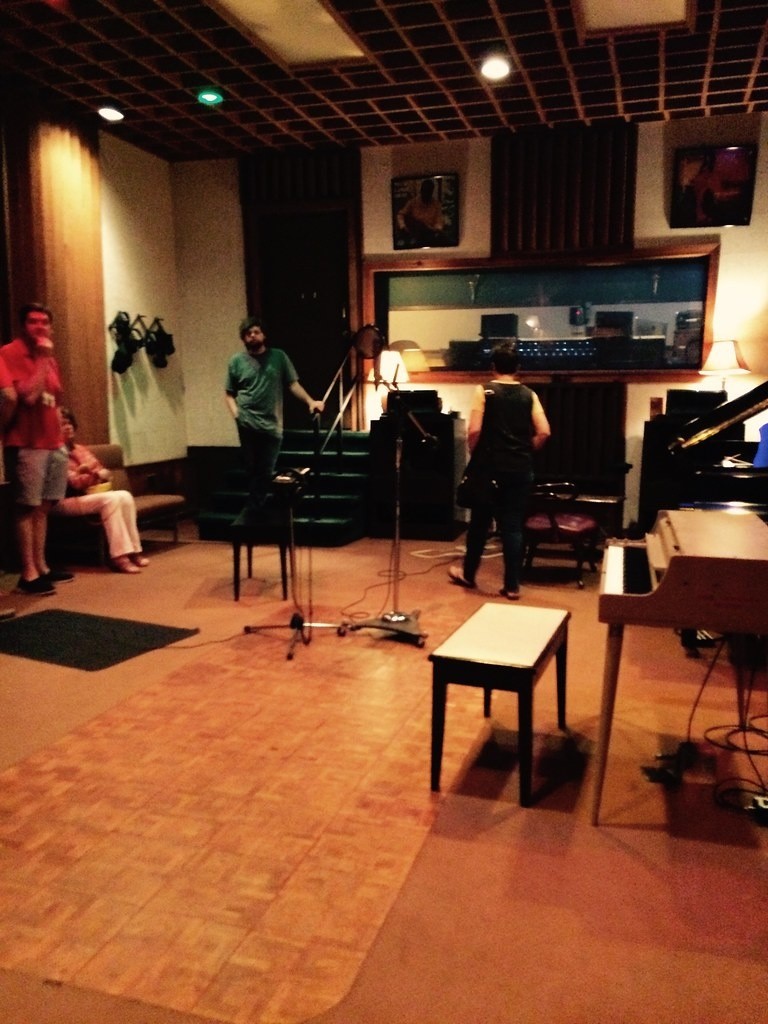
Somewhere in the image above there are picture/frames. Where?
[670,144,758,229]
[390,171,460,249]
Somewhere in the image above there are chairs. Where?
[524,482,598,589]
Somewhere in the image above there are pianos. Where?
[589,506,768,828]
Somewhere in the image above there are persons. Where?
[446,339,551,599]
[224,318,324,531]
[47,407,149,574]
[0,302,77,596]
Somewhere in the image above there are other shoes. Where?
[111,556,140,573]
[42,570,74,583]
[447,565,476,585]
[129,552,150,567]
[16,577,56,595]
[500,587,520,599]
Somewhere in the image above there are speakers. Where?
[638,390,754,540]
[365,391,470,541]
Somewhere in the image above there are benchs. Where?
[428,603,572,808]
[231,505,291,601]
[82,444,183,564]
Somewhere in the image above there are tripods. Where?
[242,376,364,661]
[338,377,435,645]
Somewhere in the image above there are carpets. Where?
[0,609,199,673]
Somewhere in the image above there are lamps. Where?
[402,349,430,372]
[698,340,751,390]
[367,349,409,414]
